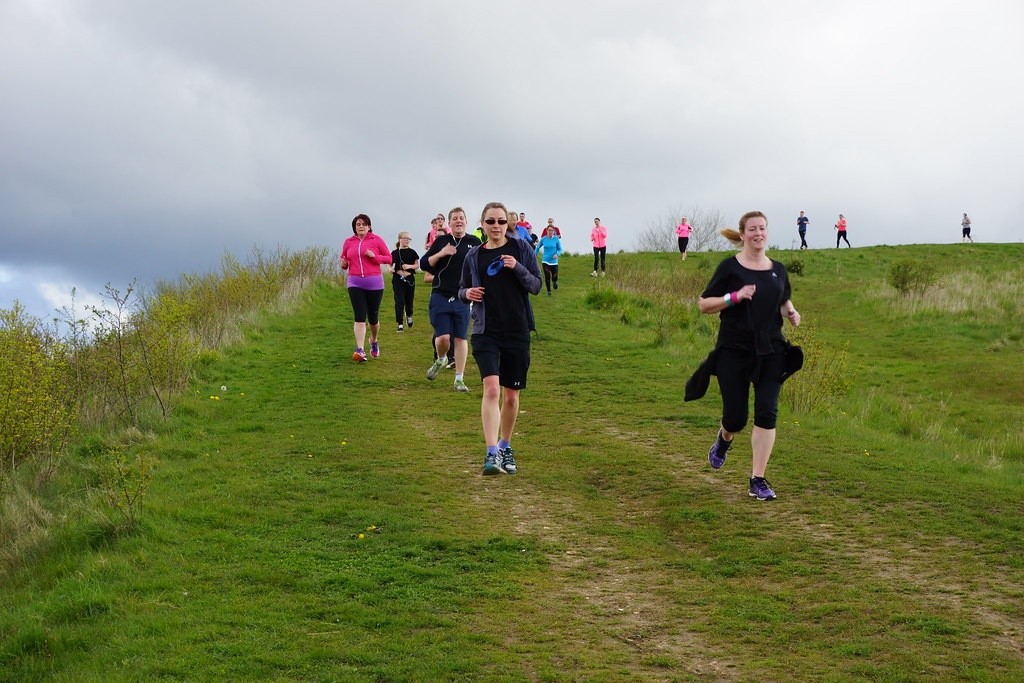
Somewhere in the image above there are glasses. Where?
[437,218,443,220]
[400,237,412,241]
[485,219,508,225]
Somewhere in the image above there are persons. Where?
[796,211,810,249]
[341,214,391,361]
[962,213,974,243]
[684,212,803,501]
[676,218,693,260]
[590,218,607,277]
[420,207,481,392]
[525,225,539,246]
[834,214,851,248]
[386,231,420,332]
[473,212,536,251]
[518,213,529,227]
[541,218,561,239]
[534,225,562,295]
[425,213,456,369]
[458,202,543,474]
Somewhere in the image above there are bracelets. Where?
[731,291,741,303]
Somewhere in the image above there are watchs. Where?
[724,293,733,305]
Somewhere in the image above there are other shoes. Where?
[553,282,558,289]
[445,358,455,369]
[547,291,551,295]
[590,271,597,277]
[601,271,606,276]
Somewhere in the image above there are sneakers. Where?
[498,440,516,472]
[482,452,501,474]
[454,379,468,392]
[369,337,380,358]
[426,357,449,381]
[748,476,777,501]
[397,325,404,333]
[708,428,734,469]
[353,348,367,362]
[405,312,413,328]
